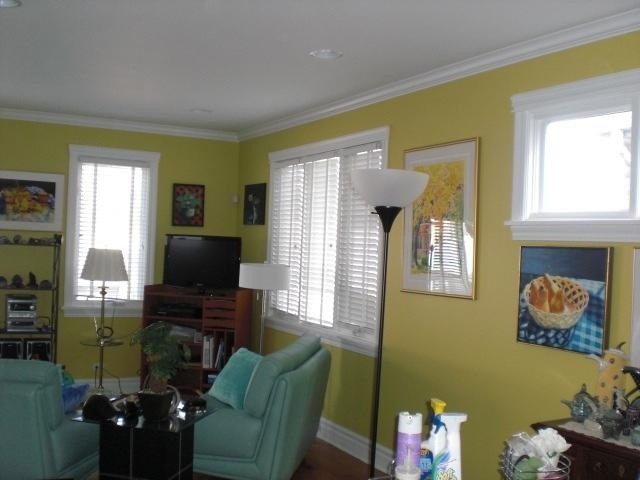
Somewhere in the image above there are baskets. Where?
[523,275,591,330]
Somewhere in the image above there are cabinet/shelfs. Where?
[140,284,253,392]
[0,234,63,365]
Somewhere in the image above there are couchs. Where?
[193,329,332,480]
[0,358,100,480]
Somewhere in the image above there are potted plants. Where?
[129,321,191,422]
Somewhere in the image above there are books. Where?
[202,335,224,369]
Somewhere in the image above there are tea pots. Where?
[560,340,640,449]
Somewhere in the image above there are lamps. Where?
[350,168,430,480]
[238,262,290,356]
[80,248,129,392]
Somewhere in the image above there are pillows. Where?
[0,358,65,431]
[207,347,264,411]
[242,330,322,417]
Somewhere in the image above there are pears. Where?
[529,274,566,314]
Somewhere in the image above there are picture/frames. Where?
[0,169,66,234]
[171,182,206,228]
[242,182,267,226]
[516,244,615,359]
[399,135,482,302]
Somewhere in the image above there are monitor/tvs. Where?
[163,233,242,297]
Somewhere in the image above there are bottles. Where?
[395,412,422,480]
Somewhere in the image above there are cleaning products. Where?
[420,397,469,480]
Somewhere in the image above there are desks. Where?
[529,416,640,479]
